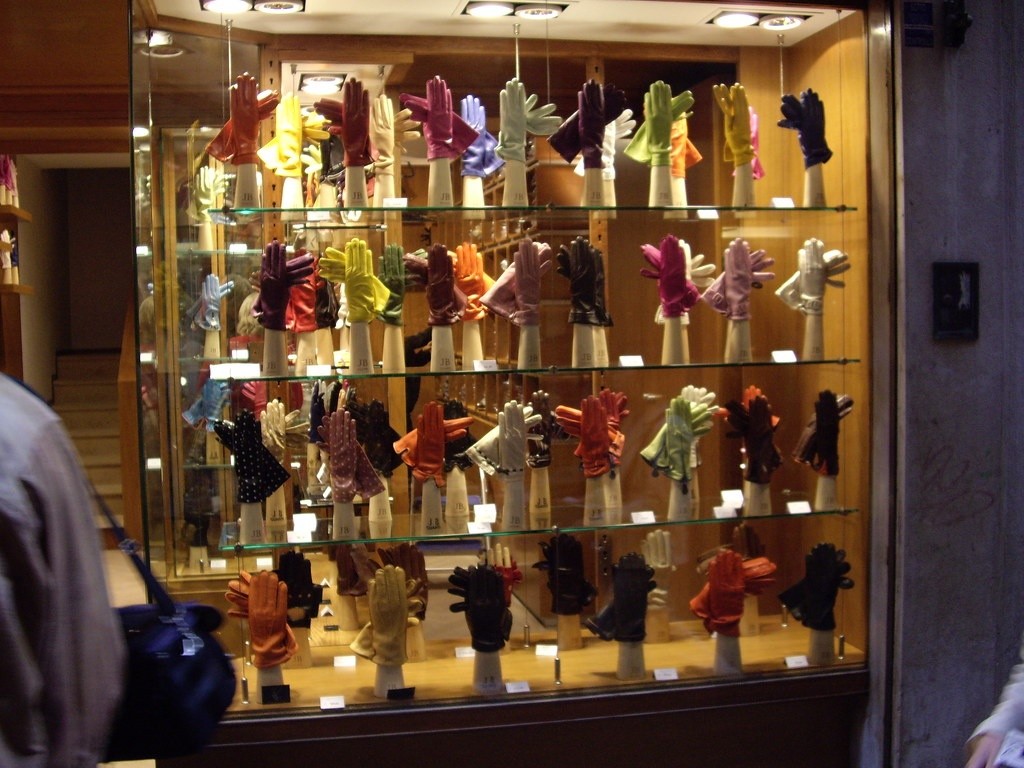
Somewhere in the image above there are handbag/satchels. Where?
[98,598,236,763]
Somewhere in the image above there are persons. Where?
[141,188,305,631]
[965,650,1024,768]
[193,234,850,376]
[225,535,858,703]
[1,371,124,767]
[181,378,855,545]
[198,74,831,209]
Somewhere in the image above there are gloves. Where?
[158,72,859,671]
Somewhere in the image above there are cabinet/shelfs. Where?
[125,0,875,768]
[0,205,37,296]
